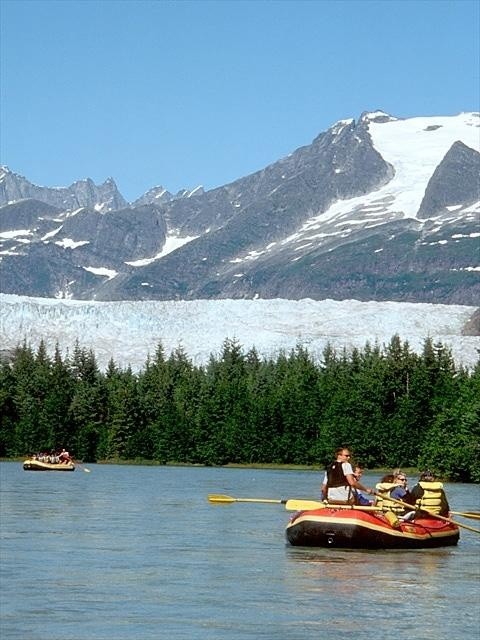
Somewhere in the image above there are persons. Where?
[320,445,453,523]
[29,446,70,465]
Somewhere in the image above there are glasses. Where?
[398,479,407,481]
[341,455,350,457]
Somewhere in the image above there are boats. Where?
[21,459,76,471]
[282,506,462,550]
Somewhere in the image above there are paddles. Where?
[285,498,405,511]
[448,510,480,521]
[66,458,91,474]
[206,493,289,506]
